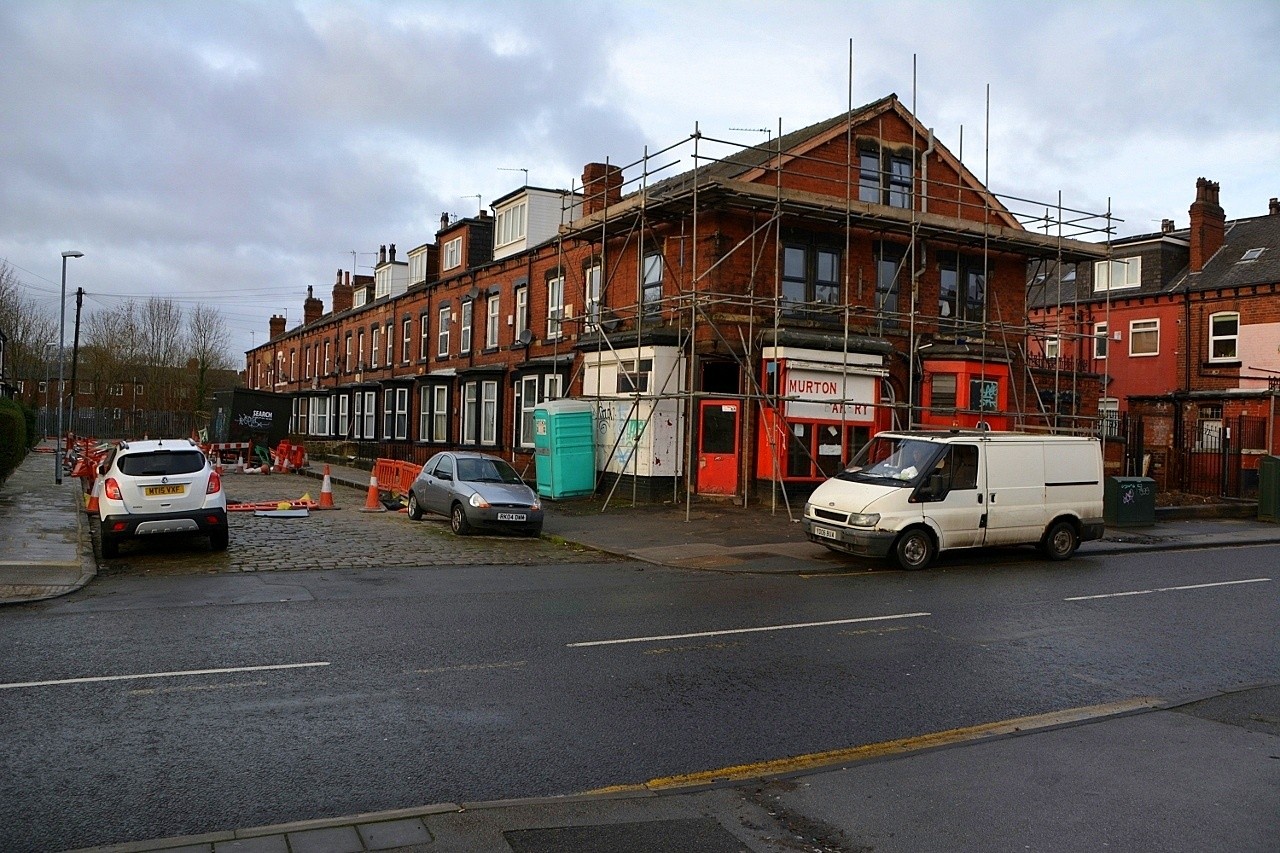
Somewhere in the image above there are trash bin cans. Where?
[1104,476,1156,527]
[1257,454,1280,524]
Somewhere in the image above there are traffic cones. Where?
[234,451,251,476]
[279,453,293,474]
[357,465,389,513]
[79,473,101,516]
[242,464,276,477]
[214,450,226,476]
[315,462,346,512]
[272,449,281,474]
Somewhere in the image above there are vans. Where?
[801,430,1106,571]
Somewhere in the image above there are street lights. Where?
[55,250,85,484]
[43,343,57,442]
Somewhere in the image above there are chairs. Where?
[939,448,977,490]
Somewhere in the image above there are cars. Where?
[405,452,544,538]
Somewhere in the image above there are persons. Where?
[889,447,926,475]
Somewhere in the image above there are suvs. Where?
[96,438,231,559]
